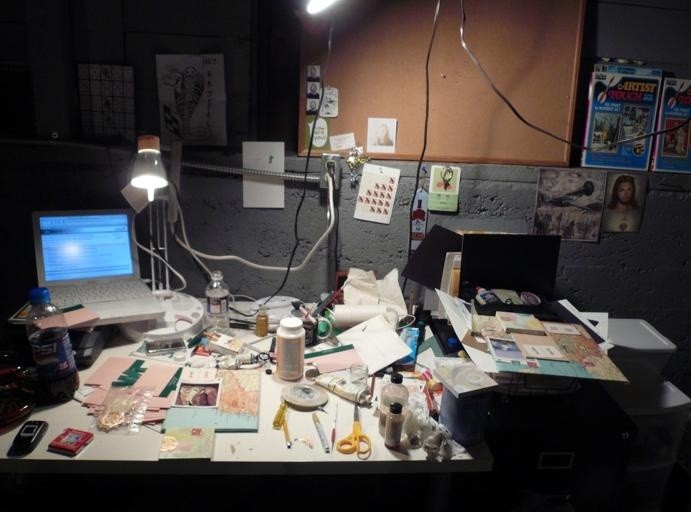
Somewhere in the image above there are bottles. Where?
[205,270,229,336]
[446,338,458,357]
[27,287,79,400]
[386,401,403,447]
[256,307,270,337]
[476,288,502,307]
[276,316,311,381]
[378,374,412,437]
[412,199,425,252]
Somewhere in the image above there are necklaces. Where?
[618,213,629,233]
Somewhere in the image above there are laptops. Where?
[32,208,166,328]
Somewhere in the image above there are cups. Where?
[289,307,315,343]
[349,361,370,388]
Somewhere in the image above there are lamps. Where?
[114,135,204,344]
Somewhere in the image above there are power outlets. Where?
[320,153,340,189]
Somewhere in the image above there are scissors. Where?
[336,402,372,454]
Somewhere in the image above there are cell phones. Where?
[13,421,49,449]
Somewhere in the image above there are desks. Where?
[0,295,493,475]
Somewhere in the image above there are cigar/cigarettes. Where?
[203,330,222,344]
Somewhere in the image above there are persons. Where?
[369,123,392,145]
[306,66,320,116]
[602,174,641,233]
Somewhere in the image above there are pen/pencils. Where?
[319,278,352,312]
[370,373,376,397]
[312,413,329,453]
[424,385,438,422]
[291,301,317,326]
[188,325,213,348]
[331,402,338,451]
[283,419,291,449]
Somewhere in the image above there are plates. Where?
[282,384,330,410]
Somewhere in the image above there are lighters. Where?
[193,346,211,355]
